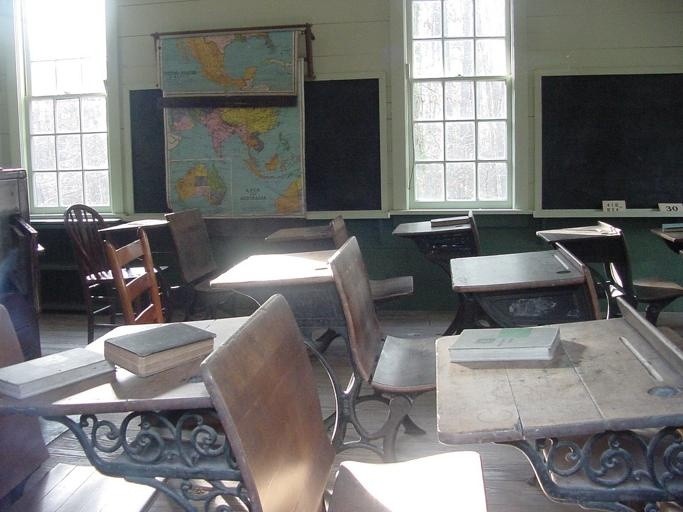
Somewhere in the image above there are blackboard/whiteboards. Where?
[123,72,387,220]
[534,68,683,218]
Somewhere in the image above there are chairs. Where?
[61,203,170,345]
[162,207,239,320]
[324,236,440,458]
[598,220,681,325]
[327,214,414,318]
[100,228,164,325]
[197,293,486,512]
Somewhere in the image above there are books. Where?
[430,216,471,227]
[451,328,562,363]
[0,346,115,401]
[104,323,213,378]
[661,222,682,233]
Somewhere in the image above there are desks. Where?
[1,314,270,511]
[209,252,334,364]
[97,219,166,232]
[433,298,682,511]
[263,221,340,244]
[441,240,601,327]
[535,221,622,264]
[391,206,491,338]
[650,219,683,257]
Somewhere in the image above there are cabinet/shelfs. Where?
[0,168,41,360]
